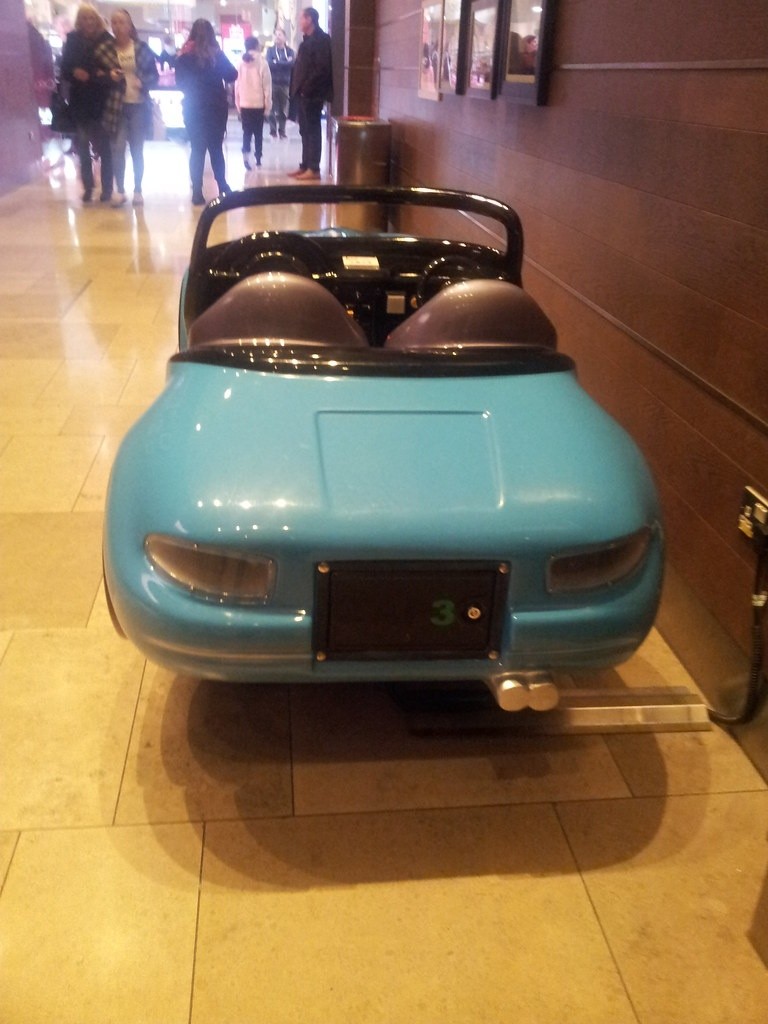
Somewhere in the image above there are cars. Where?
[101,181,664,712]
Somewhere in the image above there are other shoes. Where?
[288,169,306,177]
[244,162,251,170]
[132,192,142,206]
[279,130,286,137]
[270,131,277,137]
[220,185,234,196]
[83,188,93,200]
[192,191,205,204]
[297,168,320,179]
[112,192,126,206]
[257,159,261,165]
[100,191,113,200]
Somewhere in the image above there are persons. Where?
[286,7,335,180]
[266,28,296,139]
[160,38,181,77]
[174,18,238,204]
[421,29,538,93]
[91,7,161,208]
[234,35,273,171]
[49,83,100,160]
[60,4,118,202]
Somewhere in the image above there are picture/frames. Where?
[461,0,502,102]
[438,0,467,96]
[416,1,446,103]
[498,0,553,107]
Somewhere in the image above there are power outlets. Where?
[733,485,767,547]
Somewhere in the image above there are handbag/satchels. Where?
[51,94,78,132]
[152,101,167,140]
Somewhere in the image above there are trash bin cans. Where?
[330,113,393,234]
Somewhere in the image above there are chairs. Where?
[385,280,558,351]
[185,270,367,350]
